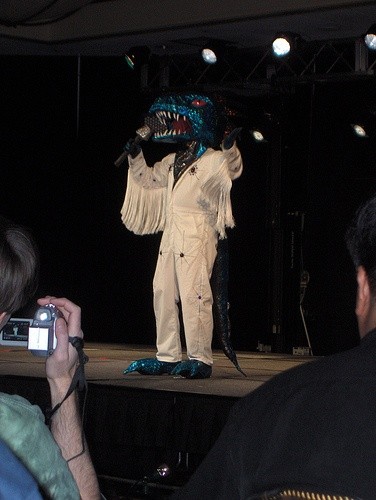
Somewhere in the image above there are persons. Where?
[116,94,243,379]
[180,195,376,500]
[0,224,103,500]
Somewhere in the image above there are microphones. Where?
[114,124,152,167]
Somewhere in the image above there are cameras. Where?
[0,303,64,358]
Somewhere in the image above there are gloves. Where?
[123,139,142,159]
[224,127,242,149]
